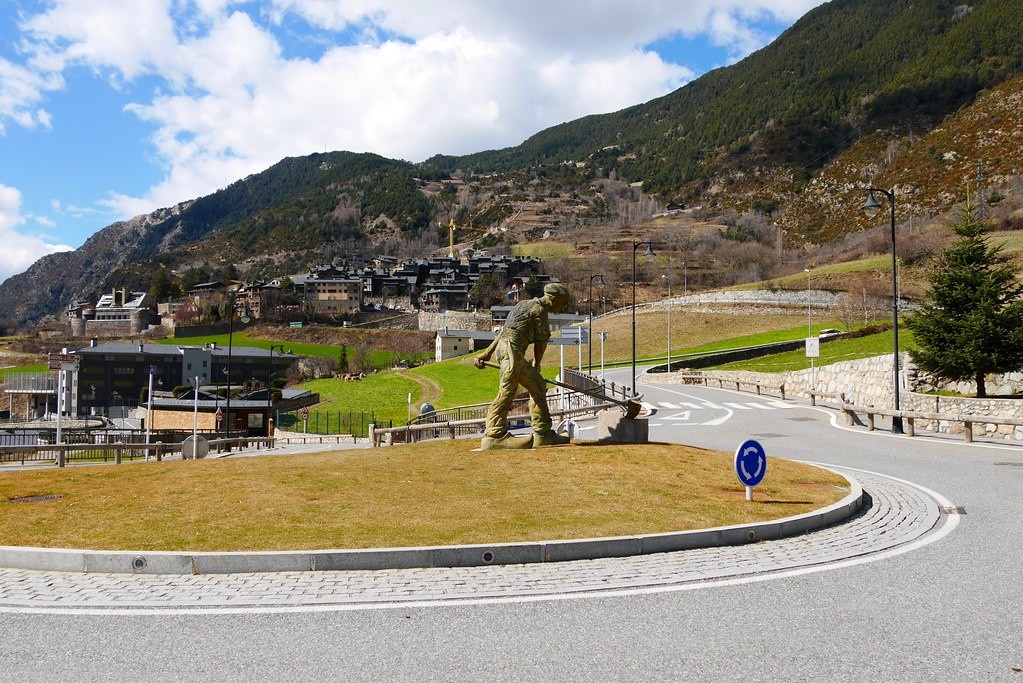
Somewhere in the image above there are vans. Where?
[819,329,844,338]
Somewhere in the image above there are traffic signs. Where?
[48,353,80,363]
[49,361,80,371]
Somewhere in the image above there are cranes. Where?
[438,219,488,257]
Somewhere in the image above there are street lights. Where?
[589,274,606,375]
[804,268,814,389]
[861,187,904,433]
[226,305,250,451]
[267,344,285,447]
[631,239,657,398]
[662,275,671,373]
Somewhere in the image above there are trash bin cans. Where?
[420,403,437,422]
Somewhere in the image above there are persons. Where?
[474,283,570,449]
